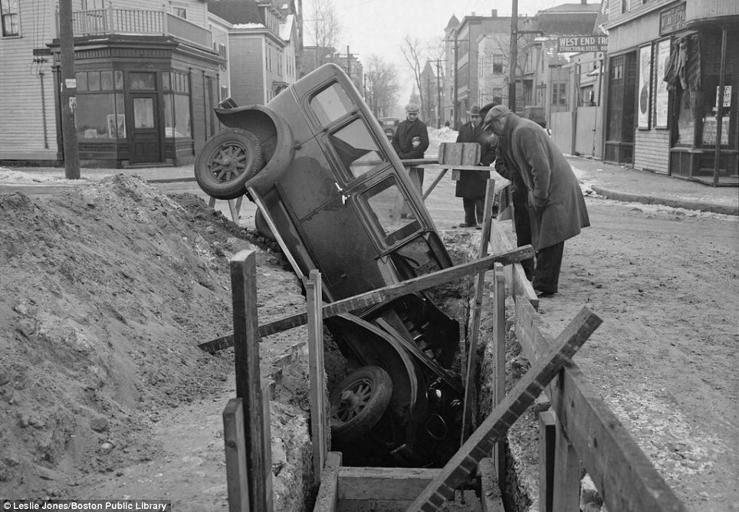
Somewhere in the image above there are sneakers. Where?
[477,216,483,224]
[534,289,554,297]
[460,218,476,227]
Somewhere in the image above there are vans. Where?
[191,62,470,461]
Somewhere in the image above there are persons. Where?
[392,103,431,220]
[477,105,535,282]
[454,106,496,230]
[481,104,592,299]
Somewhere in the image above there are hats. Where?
[465,105,481,116]
[406,104,419,114]
[478,103,497,128]
[481,104,509,132]
